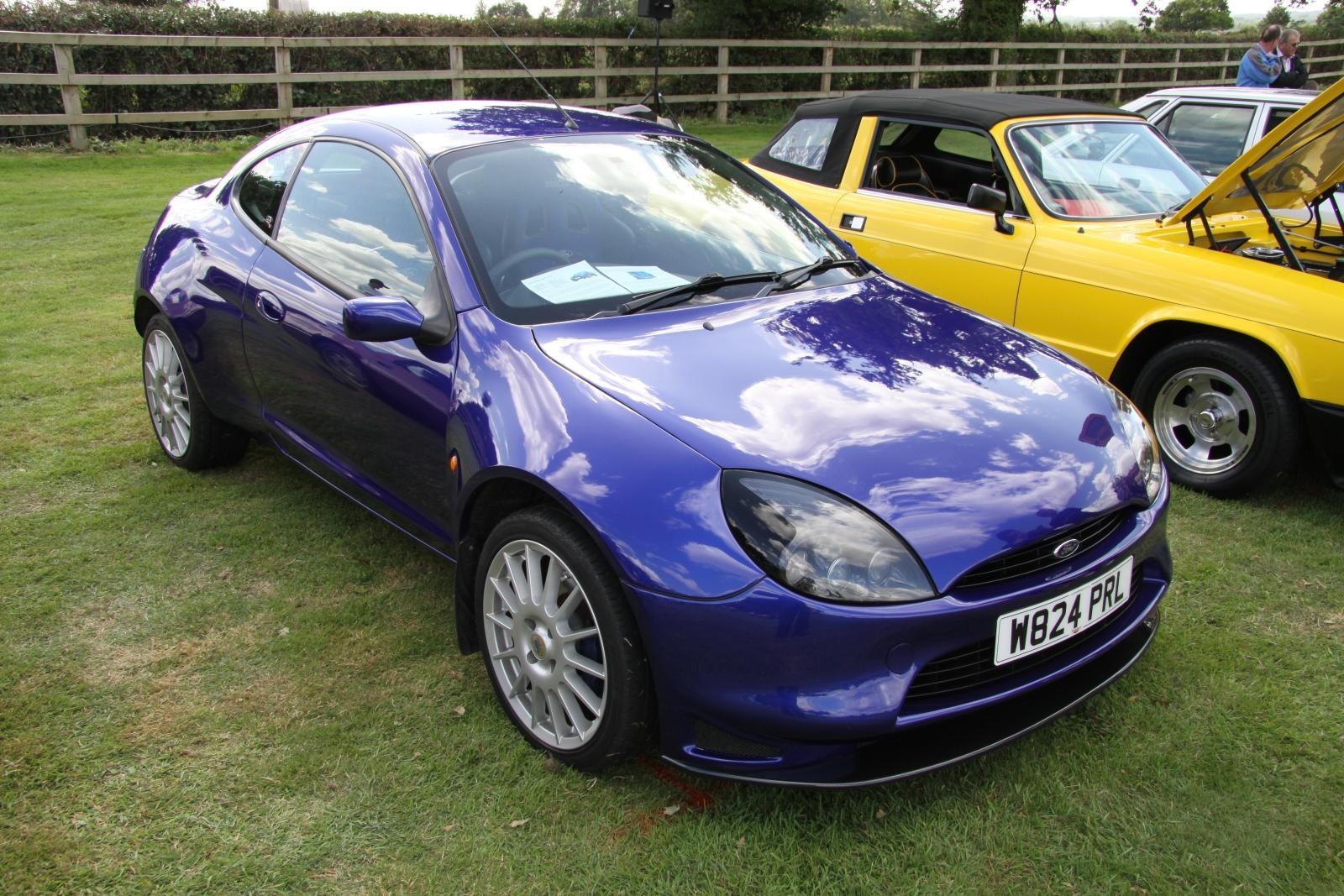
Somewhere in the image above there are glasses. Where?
[1285,41,1300,46]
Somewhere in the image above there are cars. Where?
[1031,85,1344,226]
[130,100,1180,787]
[656,77,1344,498]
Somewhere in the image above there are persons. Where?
[1270,29,1319,89]
[1236,25,1282,88]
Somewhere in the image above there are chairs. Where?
[872,154,949,200]
